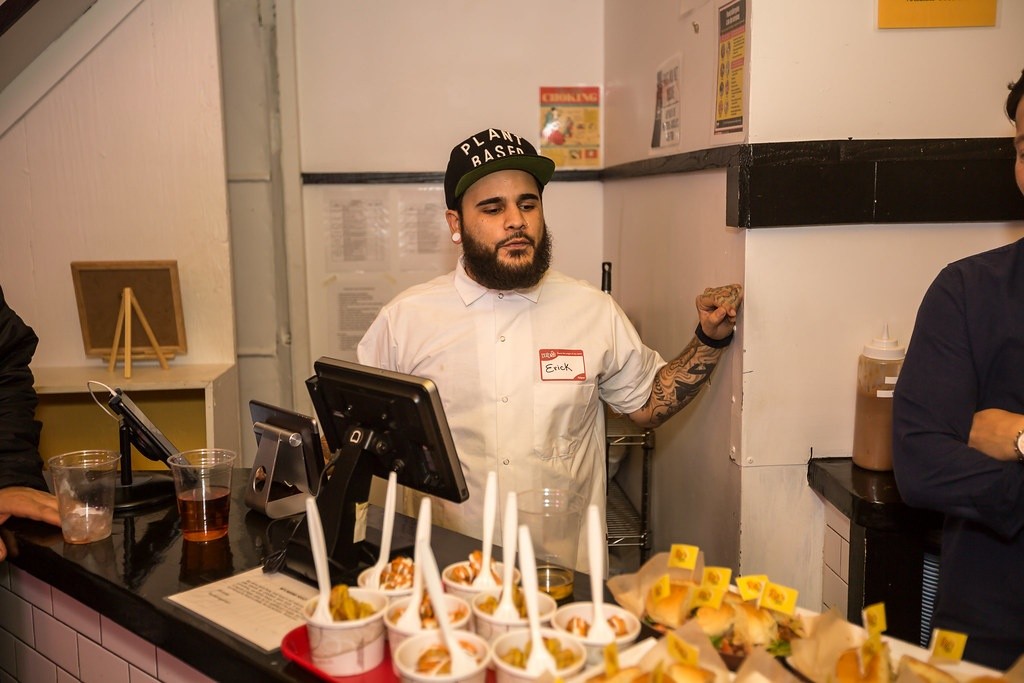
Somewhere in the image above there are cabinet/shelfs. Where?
[606,405,656,574]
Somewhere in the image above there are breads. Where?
[832,641,962,683]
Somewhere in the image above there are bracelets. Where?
[1014,430,1024,460]
[695,322,736,349]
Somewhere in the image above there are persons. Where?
[0,284,63,559]
[893,63,1024,673]
[313,128,742,581]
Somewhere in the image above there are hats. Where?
[443,128,555,207]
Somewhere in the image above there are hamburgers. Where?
[646,581,808,659]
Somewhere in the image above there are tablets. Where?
[107,392,198,483]
[249,400,327,495]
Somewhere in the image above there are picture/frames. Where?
[71,260,189,379]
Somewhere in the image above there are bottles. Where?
[852,322,906,472]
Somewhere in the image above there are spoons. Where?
[306,472,614,674]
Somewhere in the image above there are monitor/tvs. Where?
[276,356,471,588]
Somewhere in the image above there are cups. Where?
[48,449,122,545]
[514,490,586,600]
[167,448,238,542]
[305,488,642,683]
